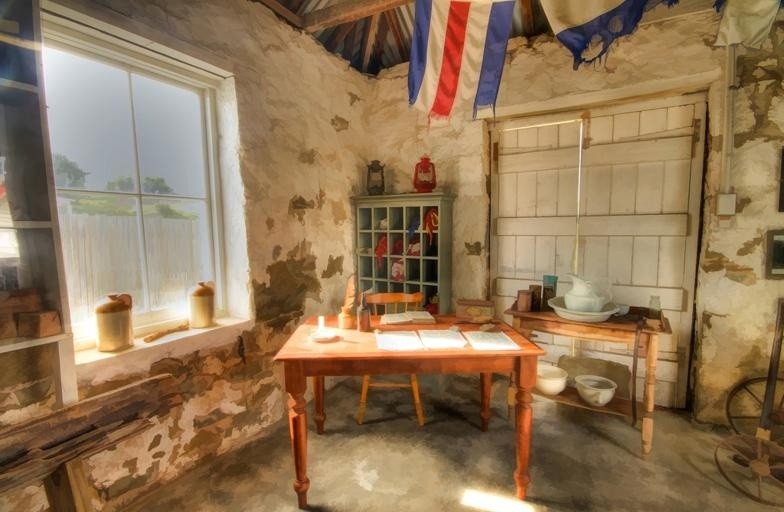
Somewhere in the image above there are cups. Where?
[518,289,532,311]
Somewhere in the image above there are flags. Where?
[539,0,680,71]
[407,1,517,122]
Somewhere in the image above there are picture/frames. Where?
[765,228,784,280]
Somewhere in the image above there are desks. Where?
[503,304,675,463]
[268,311,546,506]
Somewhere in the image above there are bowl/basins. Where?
[535,364,568,396]
[548,295,617,322]
[575,375,616,407]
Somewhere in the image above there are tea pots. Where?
[562,272,601,312]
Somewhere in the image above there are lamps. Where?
[365,159,386,195]
[413,153,436,193]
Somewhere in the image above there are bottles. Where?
[411,156,439,196]
[339,306,354,328]
[94,293,132,352]
[357,291,369,332]
[649,295,660,318]
[543,274,556,310]
[189,281,215,328]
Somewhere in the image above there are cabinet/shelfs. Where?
[349,191,459,393]
[0,1,84,412]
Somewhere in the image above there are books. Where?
[379,310,436,327]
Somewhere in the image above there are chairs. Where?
[357,289,428,429]
